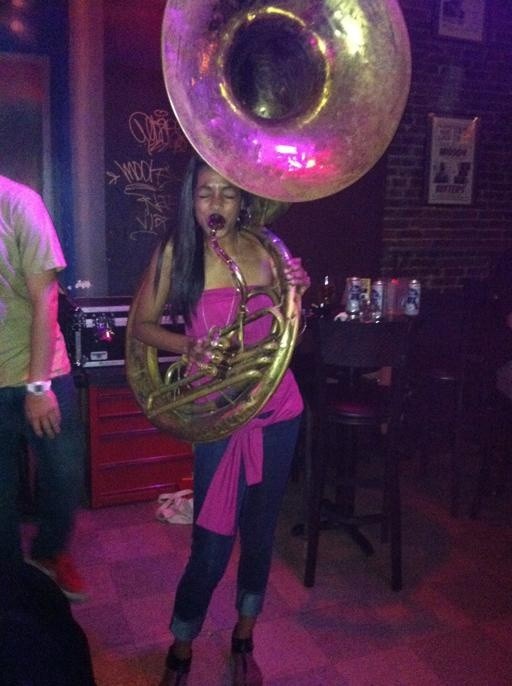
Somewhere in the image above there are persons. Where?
[453,160,471,184]
[433,160,450,185]
[128,149,312,686]
[1,172,89,603]
[1,556,94,685]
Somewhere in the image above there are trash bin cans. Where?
[23,382,54,397]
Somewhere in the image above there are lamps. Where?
[293,310,414,590]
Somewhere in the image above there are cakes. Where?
[345,276,361,314]
[370,281,385,319]
[404,279,422,316]
[384,280,400,315]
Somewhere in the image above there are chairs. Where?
[23,550,89,601]
[160,644,191,686]
[231,637,263,686]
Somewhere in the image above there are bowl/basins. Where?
[199,230,241,336]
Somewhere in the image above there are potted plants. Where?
[422,16,480,209]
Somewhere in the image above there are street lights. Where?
[156,489,193,525]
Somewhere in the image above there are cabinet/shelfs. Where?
[343,277,362,323]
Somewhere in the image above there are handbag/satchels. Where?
[297,312,309,338]
